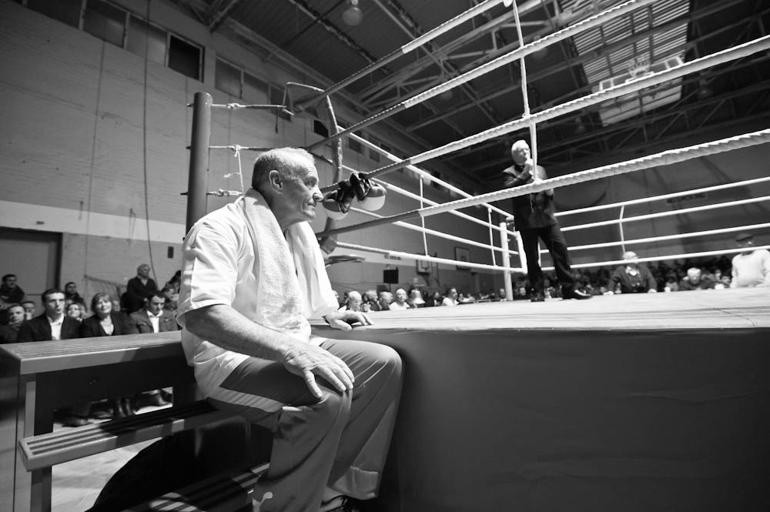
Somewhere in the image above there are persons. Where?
[1,264,185,427]
[502,139,591,302]
[178,146,409,511]
[331,234,770,313]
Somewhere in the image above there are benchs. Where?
[0,323,255,511]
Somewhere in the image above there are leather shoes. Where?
[562,288,589,300]
[529,292,545,302]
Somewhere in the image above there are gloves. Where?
[351,171,386,212]
[323,180,353,221]
